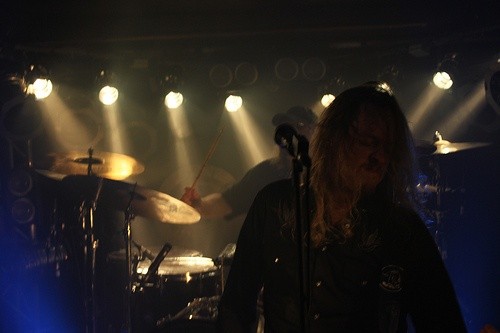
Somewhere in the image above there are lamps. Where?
[319,83,339,107]
[431,52,461,91]
[217,85,243,112]
[20,60,57,100]
[208,52,327,88]
[158,70,185,108]
[93,69,120,103]
[375,61,400,96]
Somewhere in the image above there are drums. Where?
[130,256,222,333]
[409,183,457,212]
[107,246,206,296]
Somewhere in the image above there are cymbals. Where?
[428,139,490,155]
[64,174,201,225]
[31,150,145,181]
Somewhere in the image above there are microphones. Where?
[274,124,311,168]
[132,242,172,294]
[132,241,154,261]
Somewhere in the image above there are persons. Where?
[182,106,317,219]
[217,81,467,333]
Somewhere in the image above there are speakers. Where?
[0,165,41,247]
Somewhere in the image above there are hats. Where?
[272,106,318,131]
